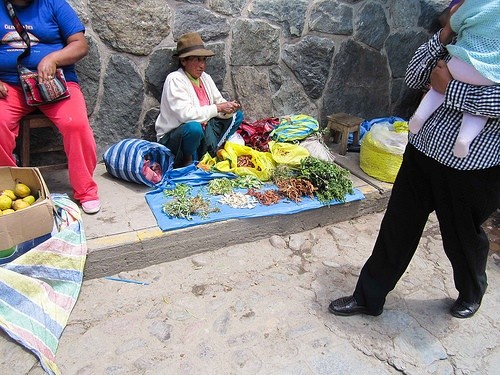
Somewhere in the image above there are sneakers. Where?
[81,199,101,214]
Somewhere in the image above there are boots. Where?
[207,150,215,158]
[180,153,193,167]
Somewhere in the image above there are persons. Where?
[155,33,243,170]
[0,0,101,214]
[408,0,500,158]
[328,0,500,318]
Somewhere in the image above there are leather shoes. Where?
[450,295,482,318]
[329,295,383,316]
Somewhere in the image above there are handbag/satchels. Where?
[18,67,71,107]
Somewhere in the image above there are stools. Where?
[18,113,70,172]
[324,112,365,155]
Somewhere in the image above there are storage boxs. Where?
[0,166,67,266]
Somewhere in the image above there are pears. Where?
[0,184,36,216]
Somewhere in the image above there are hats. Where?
[172,32,217,58]
